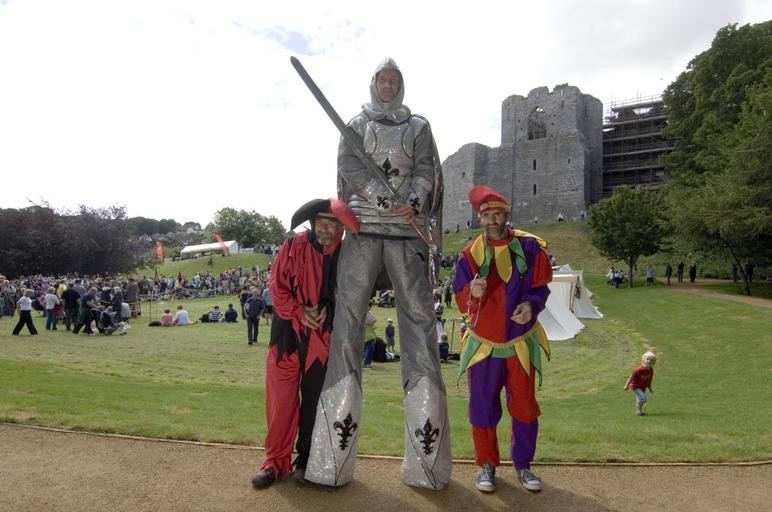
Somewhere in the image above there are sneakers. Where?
[635,410,645,416]
[251,465,279,489]
[290,467,306,481]
[516,468,542,491]
[475,461,500,493]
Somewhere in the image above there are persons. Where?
[604,260,699,288]
[253,240,284,256]
[1,263,273,346]
[307,58,453,491]
[433,255,461,360]
[449,184,555,497]
[561,213,564,221]
[534,215,540,224]
[444,221,474,234]
[743,258,753,282]
[732,264,739,283]
[580,207,586,220]
[557,211,561,222]
[549,253,556,266]
[625,351,658,417]
[248,198,359,488]
[364,289,398,366]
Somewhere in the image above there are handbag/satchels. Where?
[147,321,162,327]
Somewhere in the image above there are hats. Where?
[467,184,510,215]
[288,198,361,238]
